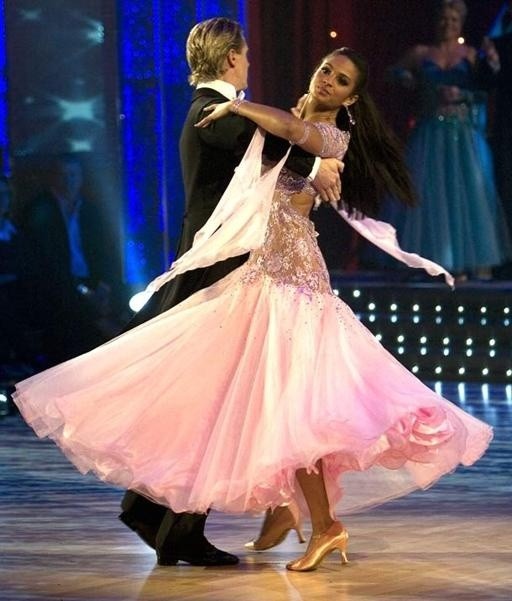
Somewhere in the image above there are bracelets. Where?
[232,97,249,114]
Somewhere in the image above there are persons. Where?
[394,0,504,281]
[117,17,347,564]
[193,46,374,572]
[13,155,118,365]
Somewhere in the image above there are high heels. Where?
[286,520,349,572]
[244,501,307,550]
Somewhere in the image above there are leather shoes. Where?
[155,547,239,566]
[118,510,156,550]
